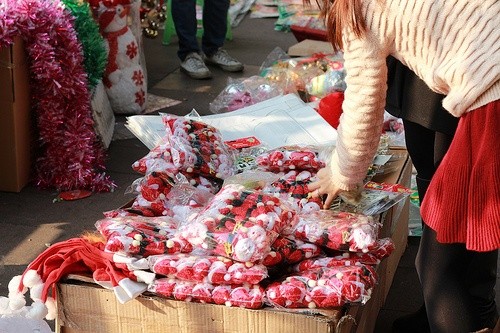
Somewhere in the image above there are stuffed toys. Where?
[96,114,395,311]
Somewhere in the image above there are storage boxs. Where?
[55,39,412,333]
[0,35,36,193]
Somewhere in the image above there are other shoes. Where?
[392,298,498,333]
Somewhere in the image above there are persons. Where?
[171,0,244,79]
[304,0,500,333]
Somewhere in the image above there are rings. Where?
[316,190,321,196]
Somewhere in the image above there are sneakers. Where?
[178,51,211,79]
[204,47,243,72]
